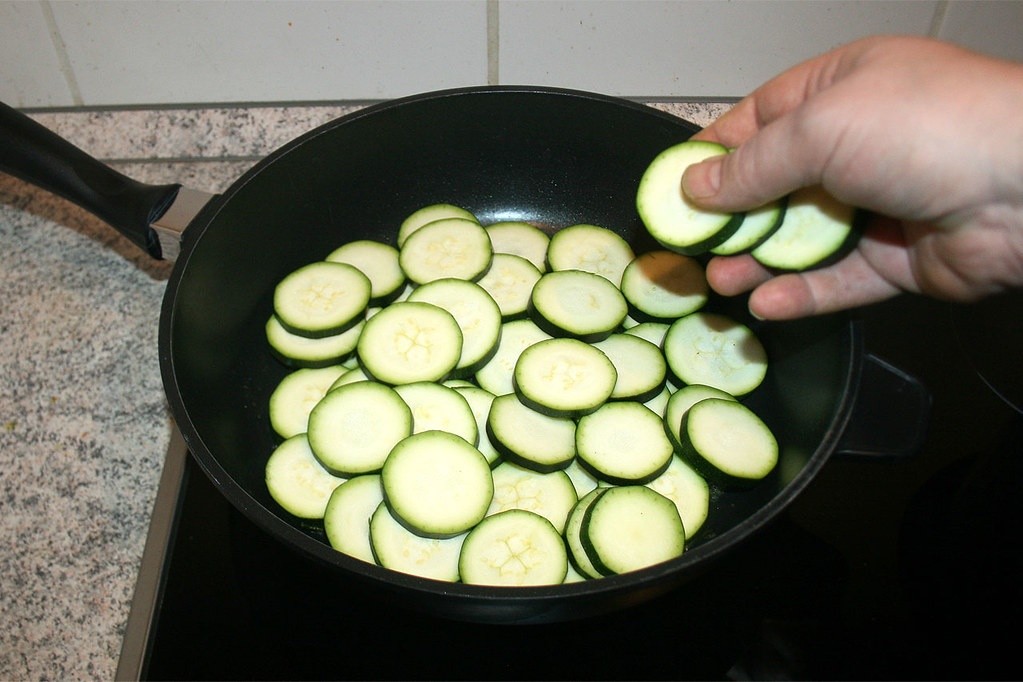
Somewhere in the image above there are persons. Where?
[675,36,1023,321]
